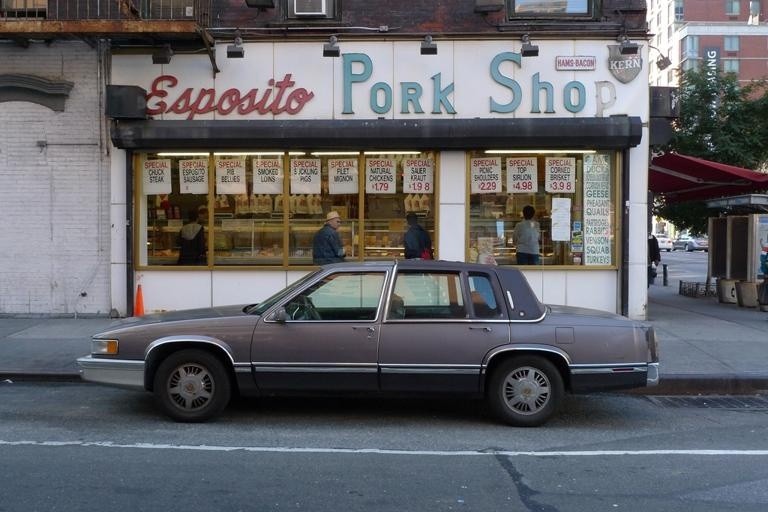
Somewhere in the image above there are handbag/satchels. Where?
[421,249,434,259]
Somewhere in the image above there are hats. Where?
[325,211,341,221]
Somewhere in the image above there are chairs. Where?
[390,295,406,319]
[464,290,492,318]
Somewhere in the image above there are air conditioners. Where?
[293,0,327,16]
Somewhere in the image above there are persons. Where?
[759,237,768,312]
[402,213,434,261]
[312,210,353,265]
[512,204,542,265]
[176,208,208,265]
[648,234,661,290]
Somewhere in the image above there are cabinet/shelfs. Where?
[153,219,523,265]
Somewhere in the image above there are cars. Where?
[76,258,657,428]
[656,232,708,252]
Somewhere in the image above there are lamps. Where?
[649,45,672,70]
[322,36,340,57]
[153,46,171,64]
[227,38,245,58]
[522,35,539,56]
[617,32,638,55]
[420,36,438,55]
